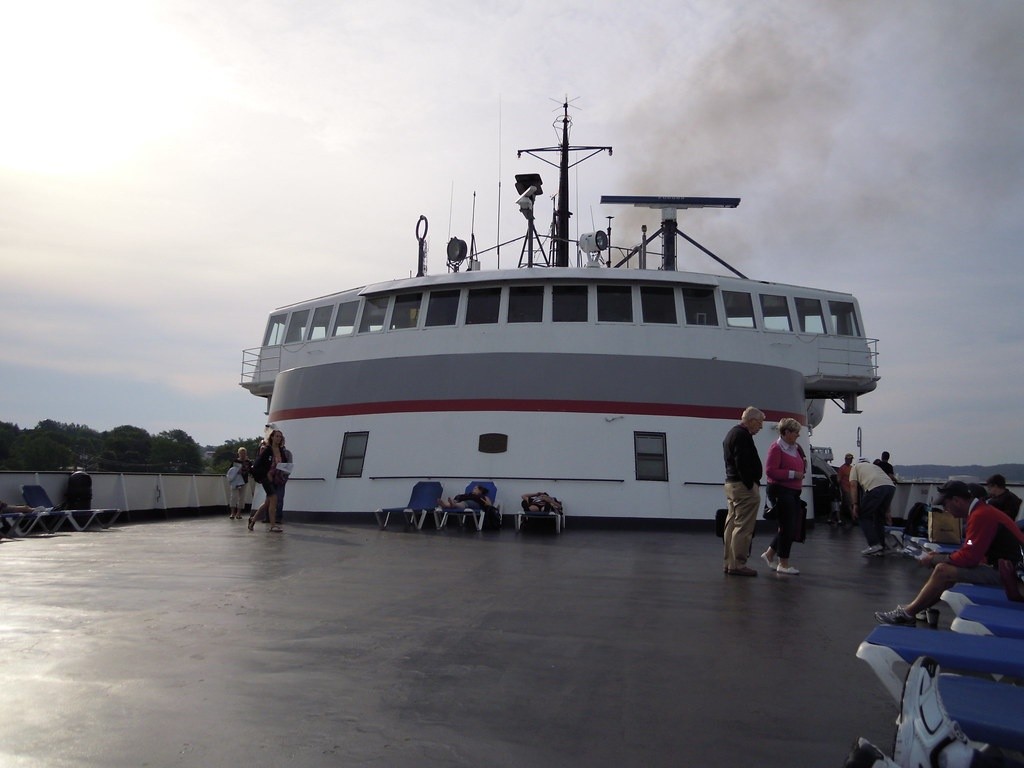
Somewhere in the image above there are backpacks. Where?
[900,502,931,541]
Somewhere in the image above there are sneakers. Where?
[841,737,903,768]
[860,543,883,556]
[869,550,884,557]
[916,610,927,621]
[874,604,917,628]
[891,654,971,768]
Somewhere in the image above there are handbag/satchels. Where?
[763,502,778,521]
[777,499,808,543]
[715,509,756,538]
[928,505,962,545]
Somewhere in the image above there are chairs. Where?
[435,481,502,533]
[0,507,71,537]
[856,516,1024,768]
[511,495,566,533]
[18,484,121,531]
[376,481,444,533]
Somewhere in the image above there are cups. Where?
[926,608,939,624]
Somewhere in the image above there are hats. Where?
[931,480,971,506]
[856,457,870,463]
[845,454,854,459]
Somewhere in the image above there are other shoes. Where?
[229,514,234,519]
[825,521,835,525]
[723,565,758,576]
[837,522,845,526]
[776,564,800,575]
[761,552,778,569]
[236,515,242,519]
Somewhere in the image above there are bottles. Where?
[35,473,39,485]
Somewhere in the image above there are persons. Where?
[825,453,860,527]
[521,492,563,515]
[723,407,765,576]
[248,430,293,532]
[0,499,66,514]
[874,480,1024,628]
[987,474,1022,522]
[437,485,492,509]
[229,447,252,519]
[848,457,896,556]
[842,656,1024,768]
[873,452,897,527]
[761,418,807,574]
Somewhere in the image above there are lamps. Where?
[446,237,467,272]
[580,230,608,267]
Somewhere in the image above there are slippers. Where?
[248,517,255,531]
[269,525,283,532]
